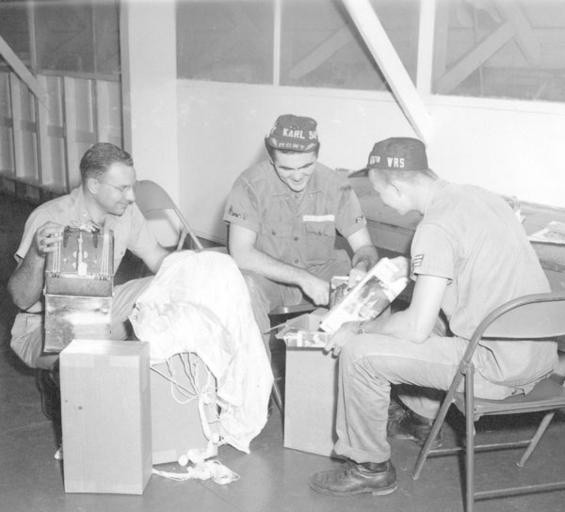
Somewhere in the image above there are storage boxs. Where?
[263,303,393,460]
[58,338,154,495]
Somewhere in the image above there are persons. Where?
[222,113,377,413]
[309,138,558,496]
[7,141,169,421]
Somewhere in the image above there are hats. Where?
[348,135,428,176]
[266,114,320,152]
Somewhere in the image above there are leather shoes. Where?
[389,408,443,450]
[310,458,398,500]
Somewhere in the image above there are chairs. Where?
[128,179,205,280]
[409,291,565,512]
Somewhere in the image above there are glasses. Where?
[101,179,132,193]
